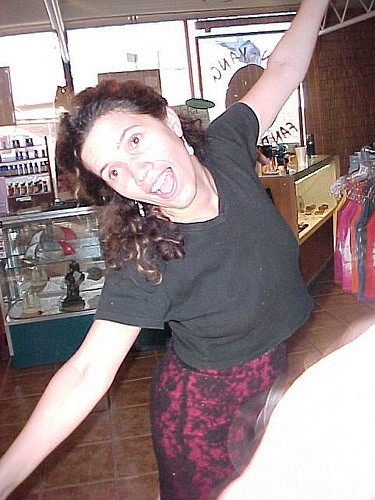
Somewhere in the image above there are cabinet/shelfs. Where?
[0,135,56,214]
[0,197,166,371]
[252,151,343,293]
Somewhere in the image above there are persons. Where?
[2,0,333,500]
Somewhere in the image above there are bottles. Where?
[0,138,49,196]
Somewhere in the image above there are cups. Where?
[295,147,307,167]
